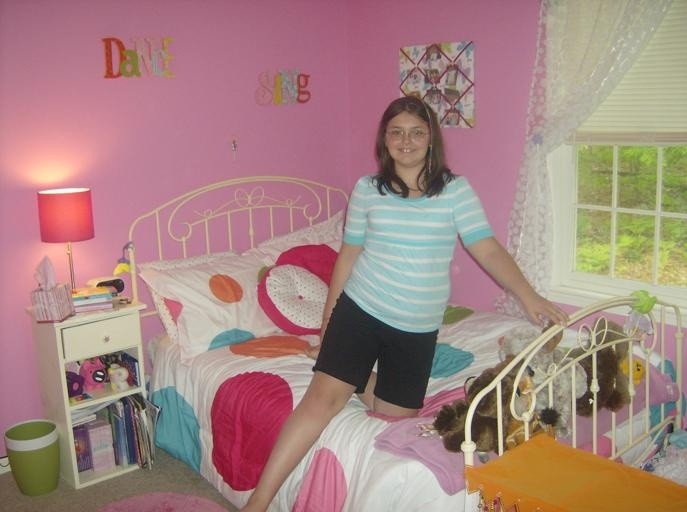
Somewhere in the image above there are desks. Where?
[464,433,687,512]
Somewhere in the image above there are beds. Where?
[128,176,683,512]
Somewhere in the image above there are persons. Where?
[239,96,571,512]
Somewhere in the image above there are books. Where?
[72,286,114,313]
[71,393,162,471]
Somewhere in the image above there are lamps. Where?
[38,188,94,294]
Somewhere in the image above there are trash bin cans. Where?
[3,420,60,496]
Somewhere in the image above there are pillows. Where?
[138,255,278,364]
[258,263,330,334]
[259,211,345,282]
[138,249,237,338]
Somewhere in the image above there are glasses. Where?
[389,129,426,139]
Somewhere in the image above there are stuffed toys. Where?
[433,320,645,454]
[65,359,129,397]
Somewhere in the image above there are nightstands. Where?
[33,301,148,490]
